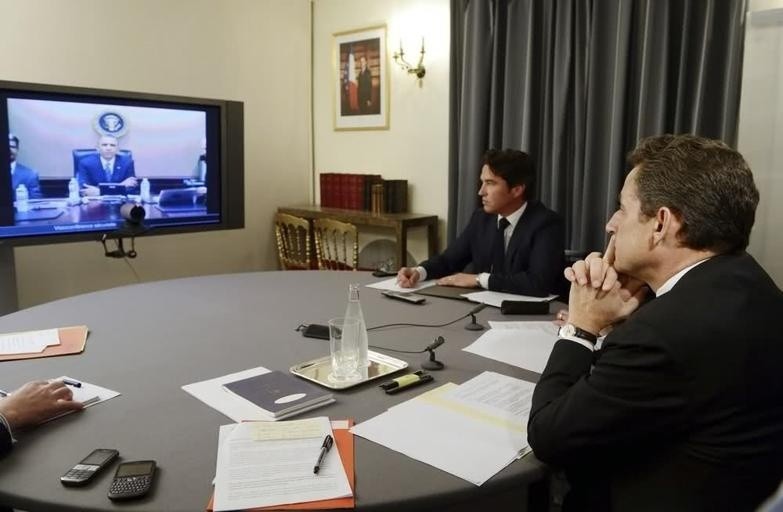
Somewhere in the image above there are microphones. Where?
[466,301,487,318]
[426,335,445,351]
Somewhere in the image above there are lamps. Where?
[388,30,430,88]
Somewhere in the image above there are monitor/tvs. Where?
[0,80,227,246]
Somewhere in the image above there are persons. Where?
[0,380,84,461]
[397,148,565,294]
[357,56,372,114]
[191,140,207,184]
[528,130,783,512]
[10,135,43,202]
[76,135,138,195]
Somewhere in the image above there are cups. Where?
[329,317,362,383]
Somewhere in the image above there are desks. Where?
[1,269,614,512]
[276,204,439,274]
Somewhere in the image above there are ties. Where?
[490,218,511,271]
[104,163,112,182]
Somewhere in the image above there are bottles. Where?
[340,283,368,365]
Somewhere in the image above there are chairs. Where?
[275,212,310,271]
[312,218,358,273]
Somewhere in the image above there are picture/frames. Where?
[329,22,391,130]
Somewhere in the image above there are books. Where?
[181,366,336,424]
[320,173,407,215]
[36,380,101,425]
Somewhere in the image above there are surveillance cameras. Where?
[99,199,146,259]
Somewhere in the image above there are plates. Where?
[290,350,408,391]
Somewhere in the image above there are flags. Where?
[344,44,358,111]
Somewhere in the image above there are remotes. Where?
[381,288,426,305]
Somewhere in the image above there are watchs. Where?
[477,273,482,286]
[558,325,597,344]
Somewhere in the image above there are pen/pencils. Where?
[312,434,335,473]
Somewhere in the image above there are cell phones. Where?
[108,459,157,504]
[59,447,119,487]
